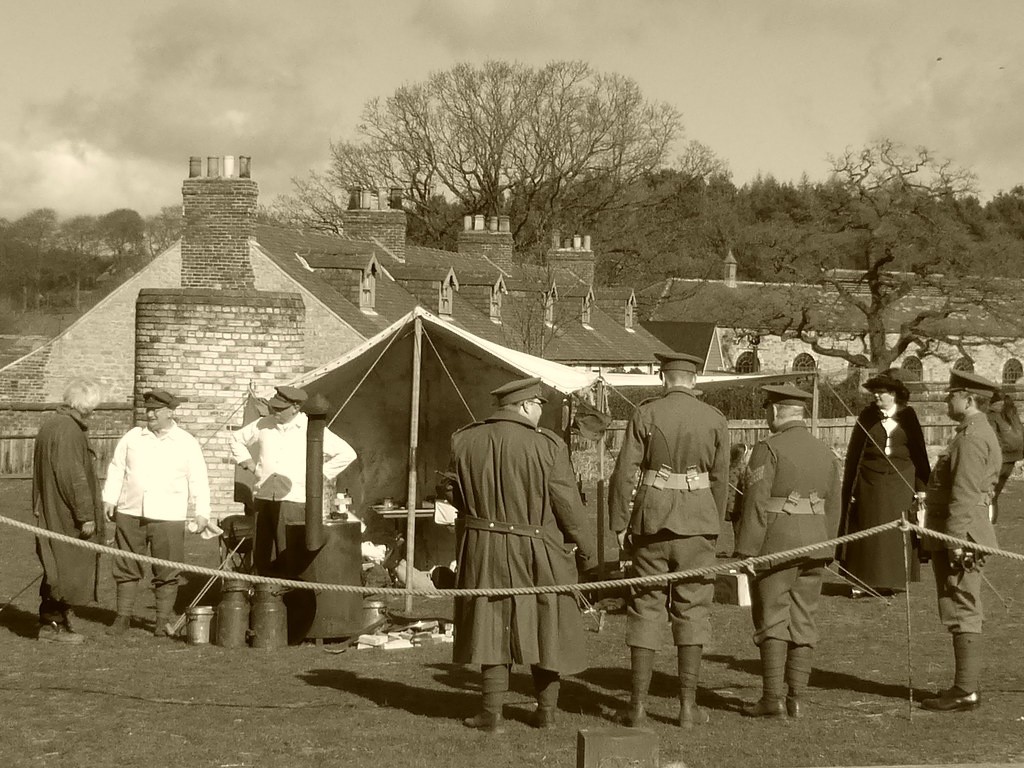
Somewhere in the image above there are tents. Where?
[237,306,824,623]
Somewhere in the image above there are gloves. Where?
[948,548,962,570]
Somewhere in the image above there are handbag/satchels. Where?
[916,504,928,540]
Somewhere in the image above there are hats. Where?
[654,352,704,380]
[491,377,549,407]
[863,377,903,391]
[761,385,813,406]
[143,390,176,408]
[269,386,308,408]
[944,367,995,397]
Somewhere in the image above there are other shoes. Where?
[155,618,169,636]
[527,708,557,731]
[106,616,130,635]
[786,697,805,718]
[742,694,787,720]
[464,708,505,734]
[38,626,85,644]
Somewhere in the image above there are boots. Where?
[621,700,646,727]
[678,687,710,728]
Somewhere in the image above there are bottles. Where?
[383,496,393,510]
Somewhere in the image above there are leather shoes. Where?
[921,692,978,712]
[938,687,981,708]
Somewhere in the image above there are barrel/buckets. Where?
[362,600,393,633]
[179,605,217,645]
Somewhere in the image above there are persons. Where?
[33,380,104,642]
[103,389,211,635]
[435,353,1024,734]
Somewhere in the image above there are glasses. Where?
[873,390,893,396]
[763,402,770,410]
[528,400,543,410]
